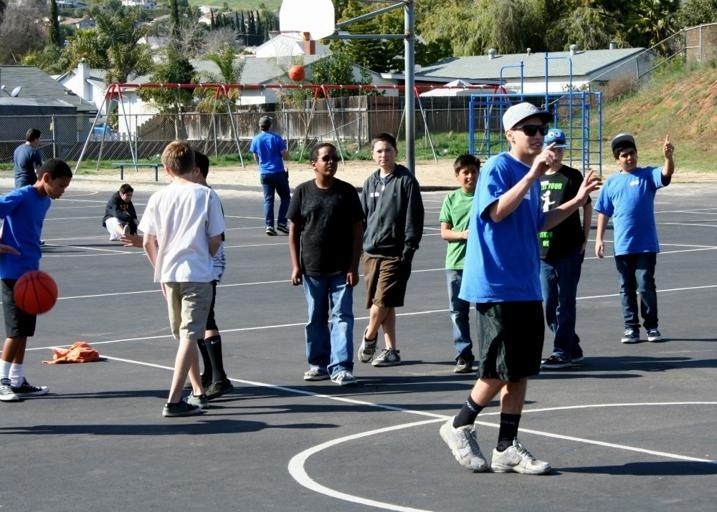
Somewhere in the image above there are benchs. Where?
[111,162,163,182]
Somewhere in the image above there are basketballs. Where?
[15,271,57,314]
[288,65,306,82]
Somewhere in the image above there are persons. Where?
[0,158,72,399]
[136,139,225,418]
[592,132,677,343]
[357,131,424,368]
[437,101,557,475]
[101,183,139,242]
[284,143,367,386]
[437,154,480,374]
[11,127,46,247]
[535,127,592,370]
[187,149,233,400]
[248,115,289,236]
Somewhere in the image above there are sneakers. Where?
[541,350,572,369]
[108,233,116,240]
[0,378,20,402]
[646,328,662,342]
[439,416,489,473]
[620,326,640,343]
[11,377,50,397]
[491,438,552,475]
[453,351,475,373]
[569,346,585,362]
[302,326,402,386]
[266,226,277,235]
[122,225,129,237]
[161,374,234,417]
[277,223,289,233]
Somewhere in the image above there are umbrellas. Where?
[416,79,515,132]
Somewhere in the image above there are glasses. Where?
[512,125,550,136]
[322,155,340,162]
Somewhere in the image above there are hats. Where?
[543,128,569,148]
[258,115,274,126]
[610,133,634,150]
[501,102,554,133]
